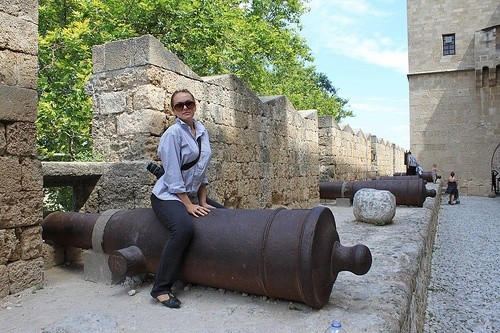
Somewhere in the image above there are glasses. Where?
[173,101,196,112]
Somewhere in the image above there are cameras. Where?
[146,163,165,179]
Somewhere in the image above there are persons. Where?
[406,152,417,176]
[150,90,229,309]
[416,164,423,178]
[448,172,459,204]
[431,163,442,182]
[492,170,499,193]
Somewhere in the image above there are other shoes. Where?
[150,292,181,308]
